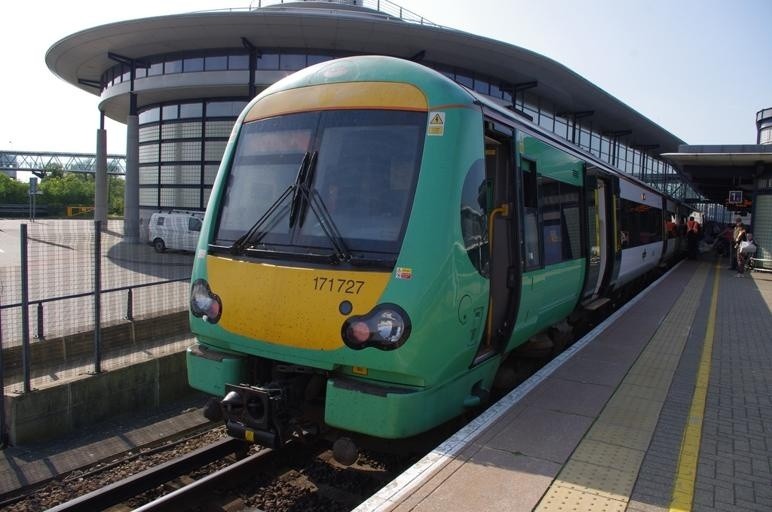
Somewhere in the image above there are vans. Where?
[147,209,204,254]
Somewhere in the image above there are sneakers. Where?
[734,272,744,278]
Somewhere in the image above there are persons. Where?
[685,216,758,278]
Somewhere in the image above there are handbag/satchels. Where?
[739,240,757,254]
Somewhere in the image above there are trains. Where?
[187,54,709,466]
